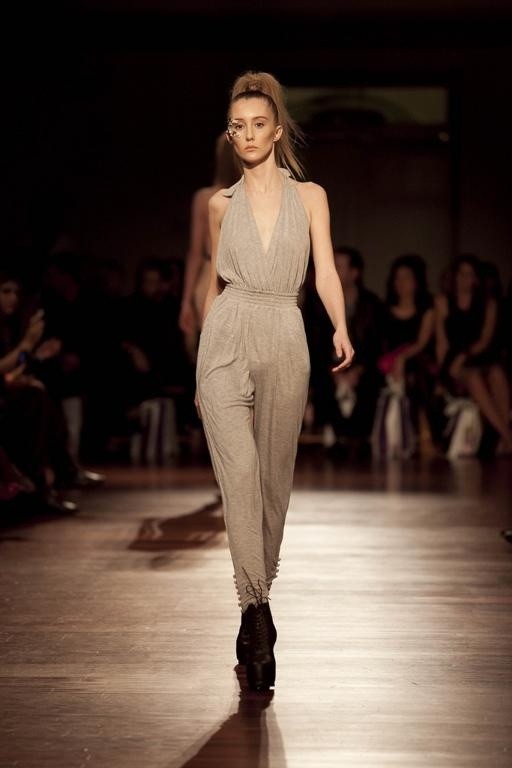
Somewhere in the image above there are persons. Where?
[192,71,356,700]
[1,234,206,522]
[297,245,510,457]
[178,125,235,497]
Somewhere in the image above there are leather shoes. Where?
[246,602,278,689]
[236,613,248,660]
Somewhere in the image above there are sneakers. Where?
[45,471,106,518]
